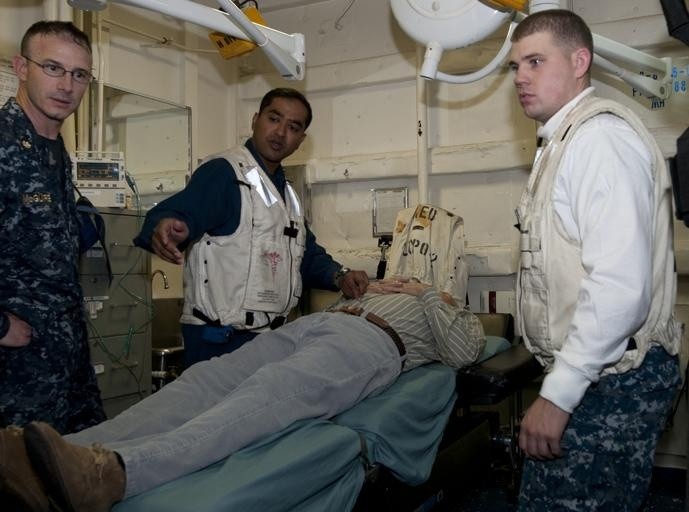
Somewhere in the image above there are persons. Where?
[1,21,106,436]
[2,275,487,512]
[133,88,369,369]
[509,9,682,512]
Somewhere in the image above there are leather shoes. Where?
[0,427,48,511]
[22,421,125,511]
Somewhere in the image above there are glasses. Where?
[22,55,95,84]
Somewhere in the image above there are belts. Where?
[325,307,405,376]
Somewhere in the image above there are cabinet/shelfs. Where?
[78,205,156,420]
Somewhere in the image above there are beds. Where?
[114,311,535,511]
